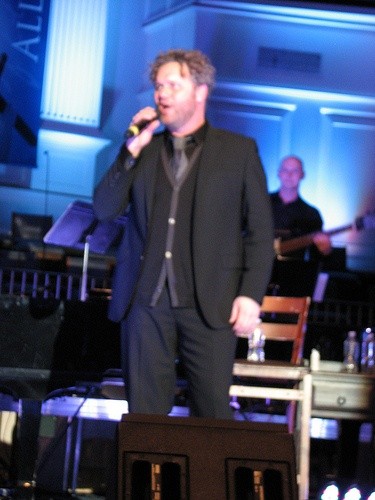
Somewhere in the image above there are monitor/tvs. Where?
[12,213,53,248]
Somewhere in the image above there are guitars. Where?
[274,209,374,258]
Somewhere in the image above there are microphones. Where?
[125,110,160,138]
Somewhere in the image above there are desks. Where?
[311,349,375,420]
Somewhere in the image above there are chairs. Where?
[232,296,310,435]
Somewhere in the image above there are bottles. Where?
[361,328,375,372]
[343,332,360,373]
[246,319,265,363]
[310,349,320,371]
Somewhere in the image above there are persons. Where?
[93,51,273,419]
[269,155,332,262]
